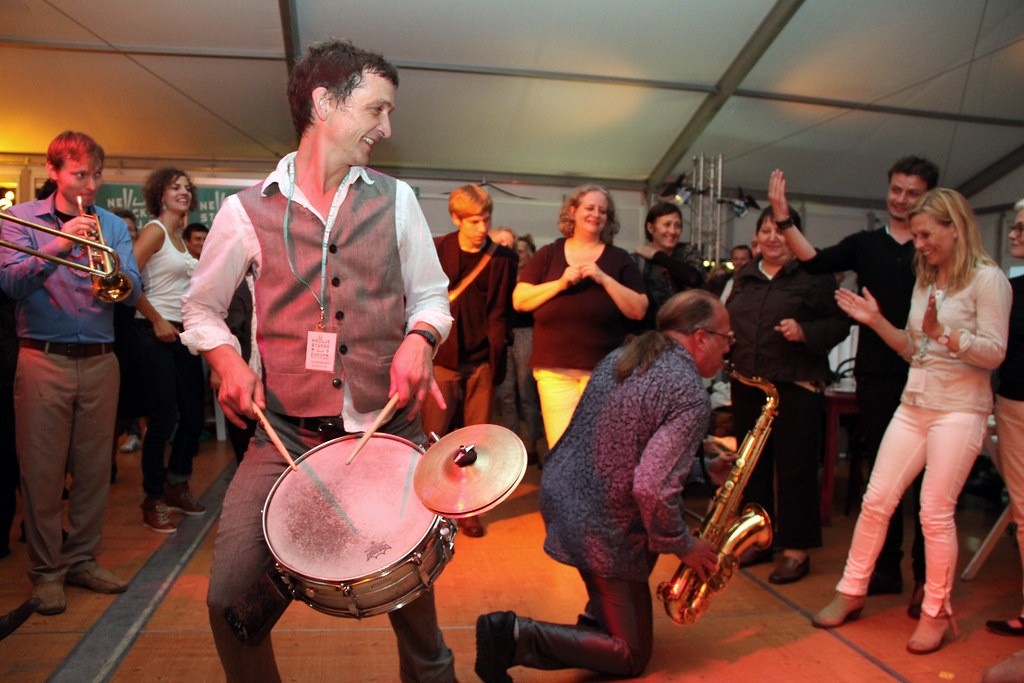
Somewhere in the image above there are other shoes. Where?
[457,516,484,537]
[986,617,1024,637]
[908,586,921,618]
[66,564,129,593]
[31,580,67,614]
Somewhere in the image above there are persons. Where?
[811,189,1012,654]
[179,35,460,683]
[422,179,853,584]
[987,198,1024,638]
[1,131,255,618]
[767,155,940,619]
[473,287,737,683]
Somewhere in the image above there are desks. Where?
[775,389,859,527]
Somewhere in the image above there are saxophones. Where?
[653,359,780,626]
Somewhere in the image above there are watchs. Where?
[405,329,437,349]
[935,325,951,345]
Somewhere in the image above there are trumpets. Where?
[76,194,131,304]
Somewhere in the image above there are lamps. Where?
[717,198,749,218]
[674,185,695,204]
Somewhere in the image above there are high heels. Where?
[907,612,949,654]
[813,593,866,627]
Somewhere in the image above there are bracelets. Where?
[772,214,797,232]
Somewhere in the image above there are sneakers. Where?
[167,492,207,515]
[141,500,177,534]
[119,435,141,452]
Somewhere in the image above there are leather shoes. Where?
[577,613,602,630]
[769,555,810,583]
[474,610,519,683]
[738,550,774,568]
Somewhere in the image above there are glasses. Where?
[692,328,734,346]
[1010,223,1024,237]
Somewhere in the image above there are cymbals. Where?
[414,424,528,518]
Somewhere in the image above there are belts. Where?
[274,413,346,438]
[19,338,115,358]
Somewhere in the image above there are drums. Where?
[261,431,456,619]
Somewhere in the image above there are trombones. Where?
[0,211,119,281]
